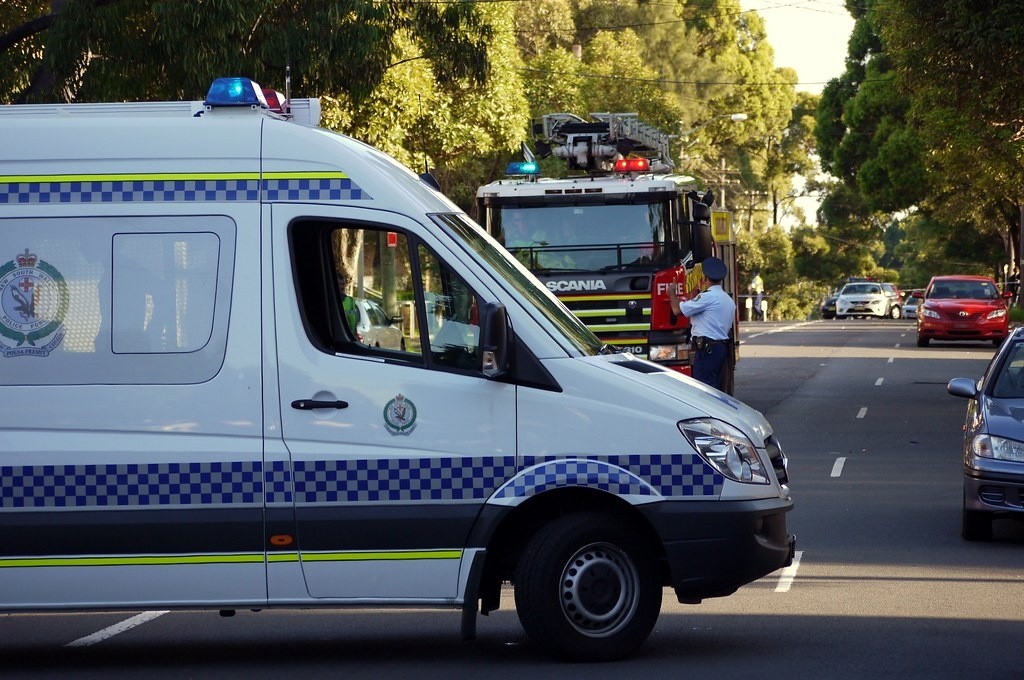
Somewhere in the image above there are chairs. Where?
[936,287,984,298]
[871,288,877,293]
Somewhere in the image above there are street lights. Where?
[667,112,748,138]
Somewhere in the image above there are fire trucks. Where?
[475,112,715,378]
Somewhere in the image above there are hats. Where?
[702,257,727,283]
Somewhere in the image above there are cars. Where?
[913,289,926,293]
[881,283,903,319]
[901,295,923,319]
[898,290,914,301]
[834,277,892,319]
[353,297,406,353]
[946,326,1024,542]
[822,292,847,319]
[912,275,1013,348]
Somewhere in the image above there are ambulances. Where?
[0,77,799,660]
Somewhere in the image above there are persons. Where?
[667,257,736,390]
